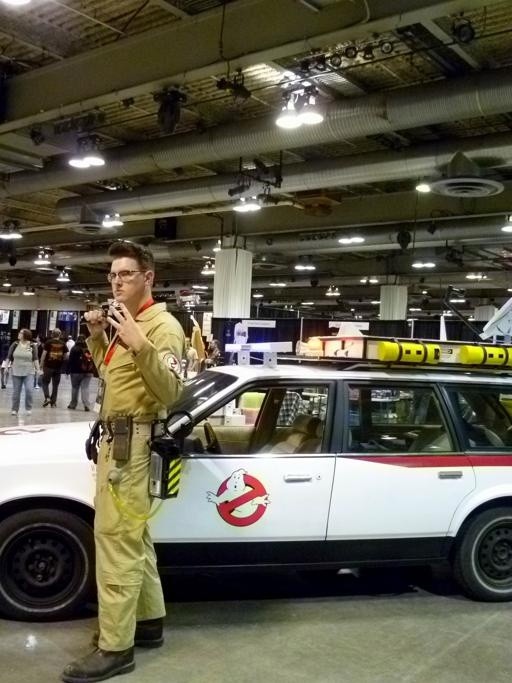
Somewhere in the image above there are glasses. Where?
[105,270,147,283]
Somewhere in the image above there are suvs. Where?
[0,336,512,622]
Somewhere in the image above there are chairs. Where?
[255,415,324,453]
[395,423,476,454]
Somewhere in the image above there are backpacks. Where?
[77,345,94,372]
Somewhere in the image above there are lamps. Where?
[68,131,106,167]
[201,258,214,276]
[275,85,325,128]
[1,249,71,295]
[0,220,23,240]
[232,173,270,213]
[102,211,124,228]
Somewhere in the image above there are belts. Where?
[99,422,163,437]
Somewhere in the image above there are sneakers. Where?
[26,410,32,416]
[61,646,135,683]
[91,616,164,650]
[42,398,57,408]
[84,406,90,411]
[35,385,41,390]
[11,409,18,416]
[1,384,6,390]
[67,405,76,410]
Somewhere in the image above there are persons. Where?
[58,241,189,682]
[0,326,223,415]
